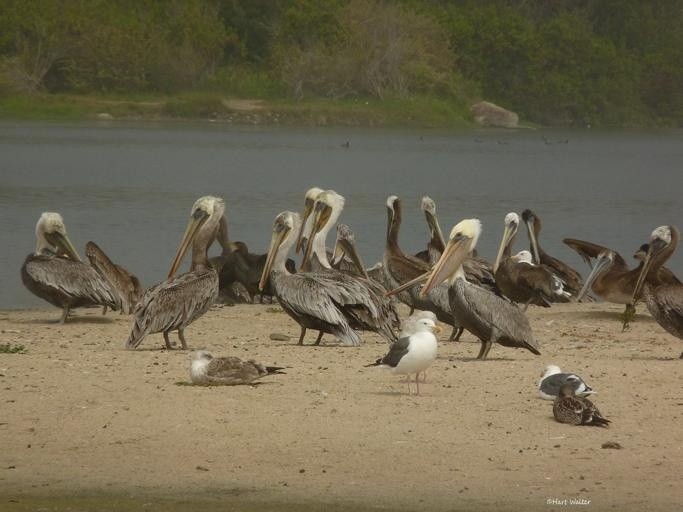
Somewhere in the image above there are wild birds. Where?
[214,188,683,360]
[191,350,287,385]
[21,211,122,325]
[86,241,143,315]
[125,194,225,349]
[553,383,613,427]
[383,318,443,394]
[538,365,597,401]
